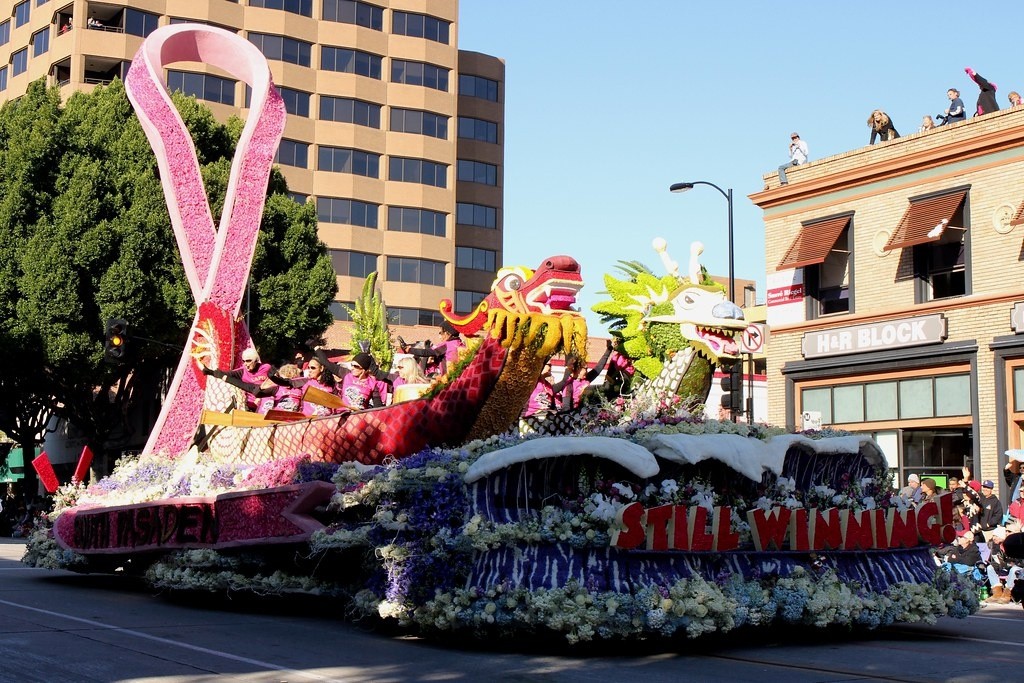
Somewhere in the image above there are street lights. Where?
[671,180,736,423]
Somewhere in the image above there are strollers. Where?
[973,538,1009,593]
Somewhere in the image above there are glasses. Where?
[438,331,446,334]
[241,359,252,363]
[350,363,361,369]
[307,366,316,370]
[398,366,404,369]
[1018,467,1024,469]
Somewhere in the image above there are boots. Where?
[984,583,1012,604]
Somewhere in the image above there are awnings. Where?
[882,192,967,251]
[776,216,851,271]
[1012,200,1024,224]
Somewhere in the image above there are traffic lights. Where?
[105,318,127,366]
[718,361,743,416]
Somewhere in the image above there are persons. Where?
[1008,92,1024,107]
[0,485,54,537]
[867,110,901,147]
[918,89,966,131]
[965,68,1000,117]
[521,336,636,418]
[196,322,466,419]
[778,133,809,187]
[899,464,1023,606]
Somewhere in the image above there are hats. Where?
[352,352,371,371]
[924,478,936,490]
[1018,486,1024,490]
[992,527,1006,538]
[439,321,460,336]
[1005,522,1021,533]
[981,480,994,487]
[955,529,974,541]
[907,473,919,483]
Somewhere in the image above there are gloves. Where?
[203,364,224,379]
[267,367,291,386]
[396,335,407,354]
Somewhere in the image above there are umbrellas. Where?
[1004,449,1024,463]
